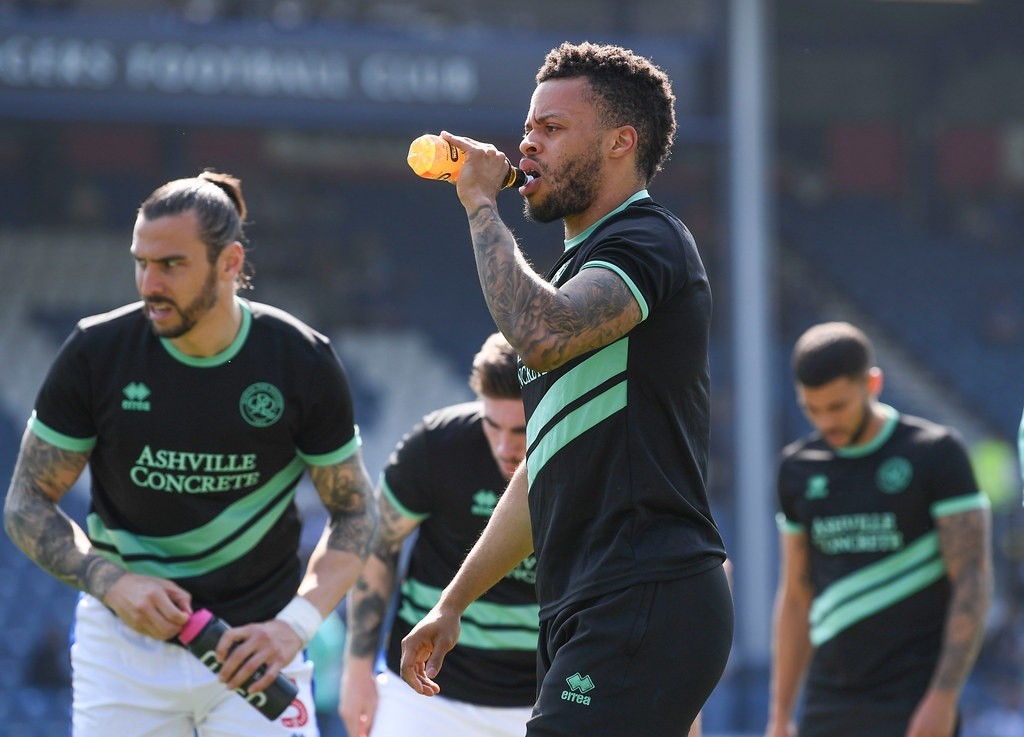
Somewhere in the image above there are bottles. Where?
[407,133,534,191]
[178,608,298,722]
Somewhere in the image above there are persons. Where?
[400,41,734,736]
[4,172,381,737]
[765,321,993,737]
[338,332,701,736]
